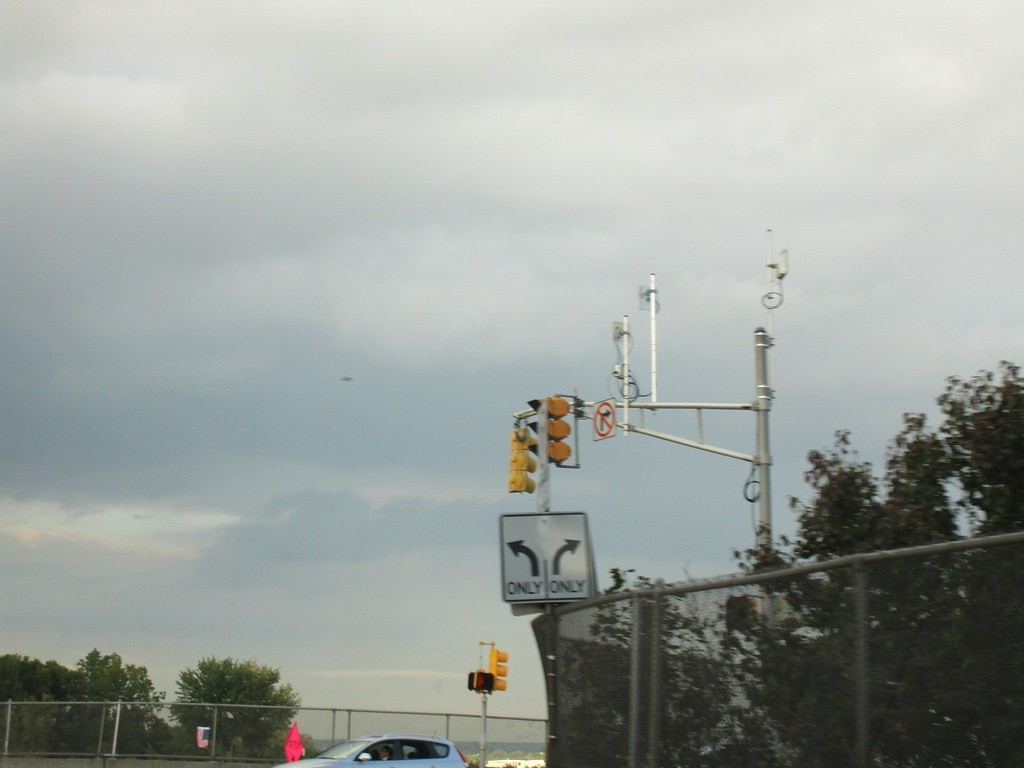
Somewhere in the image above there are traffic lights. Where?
[489,649,508,691]
[507,429,537,495]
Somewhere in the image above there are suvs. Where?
[274,733,468,768]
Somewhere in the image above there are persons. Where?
[379,746,393,761]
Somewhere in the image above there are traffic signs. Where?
[501,513,599,604]
